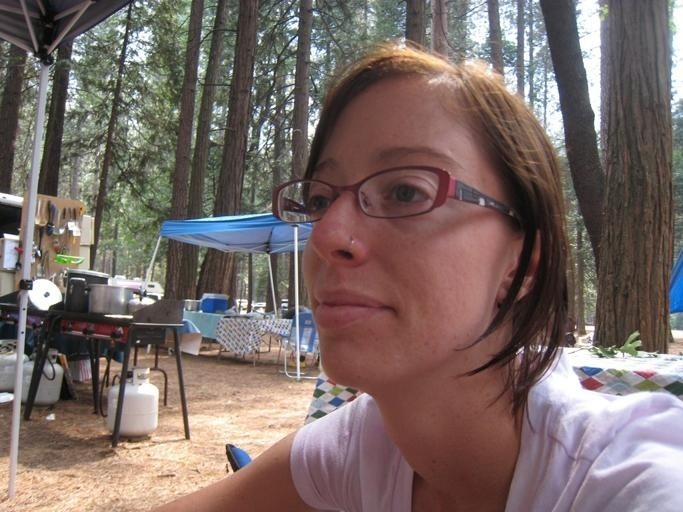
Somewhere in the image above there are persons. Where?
[149,48,683,512]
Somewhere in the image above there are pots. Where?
[184,299,200,312]
[64,269,110,312]
[85,284,139,314]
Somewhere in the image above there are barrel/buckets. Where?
[185,300,201,311]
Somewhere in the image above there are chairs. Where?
[276,310,323,379]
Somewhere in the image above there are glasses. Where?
[270,165,526,224]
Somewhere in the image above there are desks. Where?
[518,346,683,401]
[181,310,294,367]
